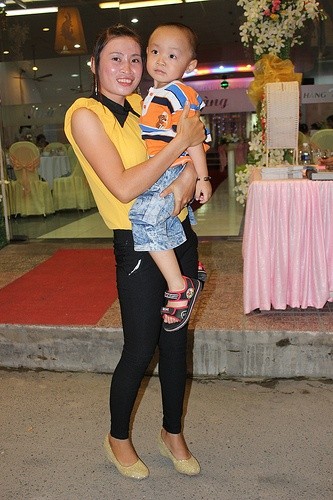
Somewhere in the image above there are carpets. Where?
[0,248,117,326]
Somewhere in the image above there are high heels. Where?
[157,429,201,476]
[103,434,149,481]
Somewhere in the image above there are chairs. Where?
[297,129,333,152]
[0,142,97,218]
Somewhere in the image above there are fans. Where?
[13,59,53,82]
[71,56,92,94]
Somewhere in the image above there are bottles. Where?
[299,143,312,165]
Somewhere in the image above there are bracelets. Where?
[195,176,211,185]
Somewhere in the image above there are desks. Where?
[241,165,333,316]
[6,155,72,190]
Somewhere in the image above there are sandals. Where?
[197,261,206,292]
[161,276,202,333]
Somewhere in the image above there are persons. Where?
[129,17,213,335]
[299,114,333,136]
[35,133,49,149]
[62,22,202,482]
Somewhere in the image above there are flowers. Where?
[234,0,329,207]
